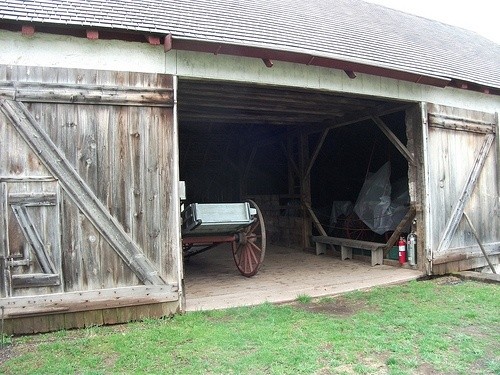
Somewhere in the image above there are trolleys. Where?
[181,198,267,278]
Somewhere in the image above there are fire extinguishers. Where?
[407,230,417,266]
[398,236,406,264]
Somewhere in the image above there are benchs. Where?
[312,236,387,267]
